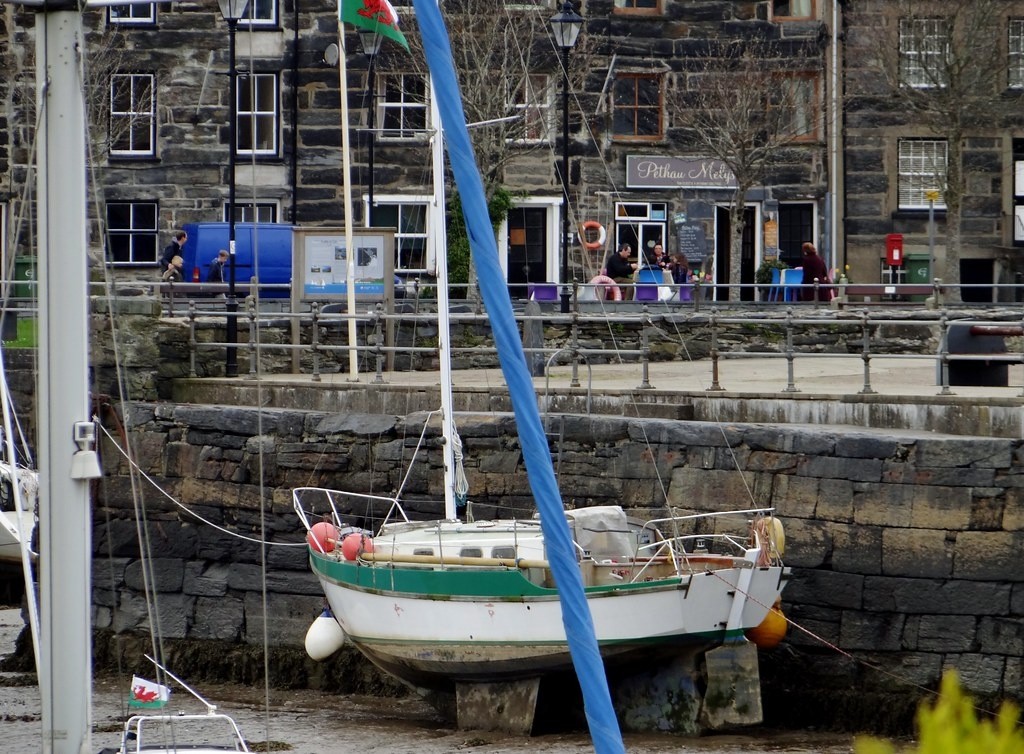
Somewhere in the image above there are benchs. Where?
[830,278,941,310]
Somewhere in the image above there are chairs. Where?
[598,264,692,301]
[767,267,835,301]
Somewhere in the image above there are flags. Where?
[339,0,412,56]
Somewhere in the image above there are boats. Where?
[0,459,41,564]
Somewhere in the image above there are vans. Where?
[180,223,406,302]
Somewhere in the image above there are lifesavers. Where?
[756,516,786,559]
[588,275,622,301]
[576,220,607,249]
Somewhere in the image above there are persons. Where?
[640,256,663,271]
[669,253,687,283]
[654,244,671,268]
[607,244,637,300]
[208,250,229,297]
[802,242,828,284]
[165,256,185,298]
[160,231,188,273]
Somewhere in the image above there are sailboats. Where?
[291,65,797,733]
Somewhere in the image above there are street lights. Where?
[549,4,585,310]
[358,24,383,223]
[219,1,250,379]
